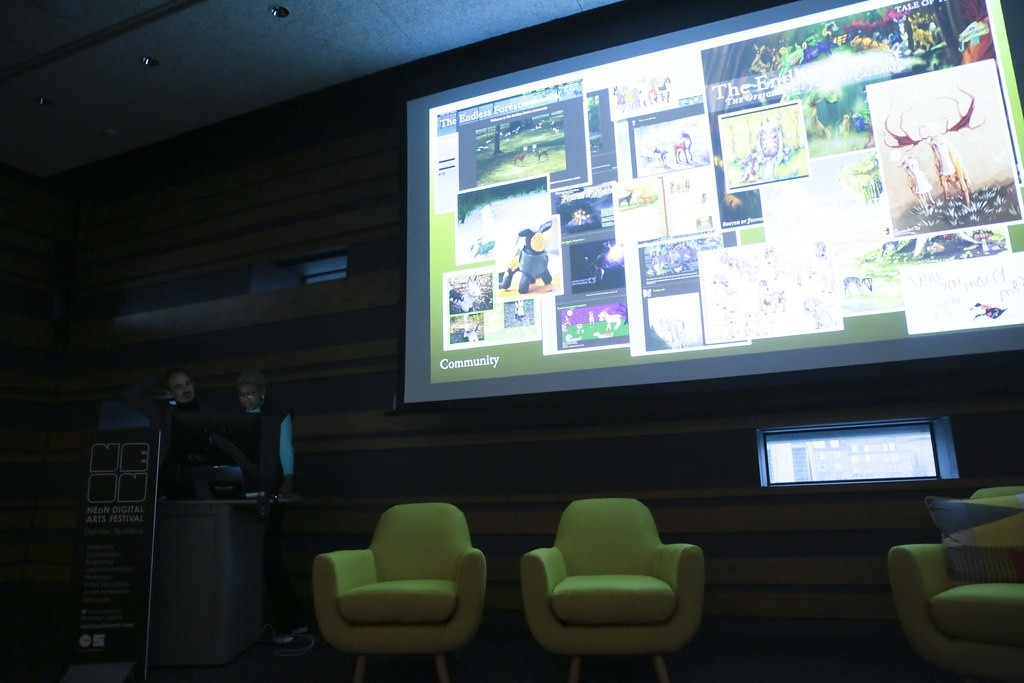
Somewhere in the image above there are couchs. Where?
[888,486,1023,683]
[312,502,487,683]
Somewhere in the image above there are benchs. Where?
[520,497,706,683]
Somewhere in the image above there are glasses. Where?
[237,391,260,399]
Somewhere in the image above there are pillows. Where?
[924,492,1023,591]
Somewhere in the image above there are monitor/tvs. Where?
[171,406,249,467]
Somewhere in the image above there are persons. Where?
[149,365,225,502]
[210,361,307,645]
